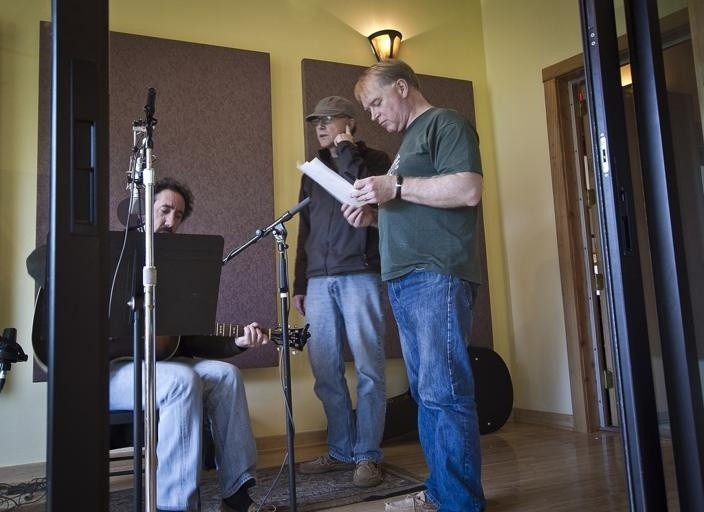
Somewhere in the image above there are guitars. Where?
[31,285,310,375]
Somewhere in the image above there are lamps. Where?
[368,29,402,64]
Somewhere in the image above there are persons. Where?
[293,94,391,485]
[107,178,270,512]
[338,59,486,511]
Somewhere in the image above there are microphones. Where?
[260,196,312,235]
[127,158,146,215]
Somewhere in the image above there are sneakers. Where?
[218,498,264,512]
[299,456,383,487]
[384,491,437,512]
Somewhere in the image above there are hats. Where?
[305,96,358,122]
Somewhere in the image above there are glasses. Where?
[310,116,343,126]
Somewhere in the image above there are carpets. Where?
[107,455,429,512]
